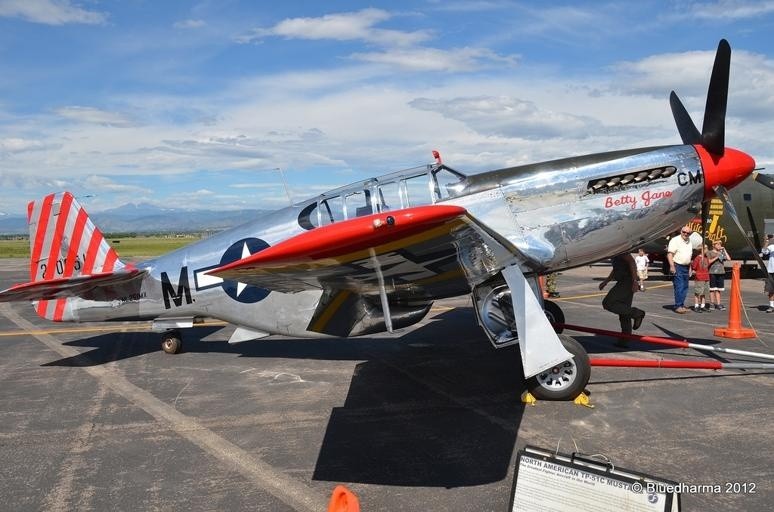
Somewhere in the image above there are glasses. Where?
[682,230,689,235]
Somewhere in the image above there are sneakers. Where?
[640,284,645,292]
[674,303,726,313]
[765,306,774,313]
[633,310,646,330]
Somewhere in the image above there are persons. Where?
[635,249,650,293]
[599,253,645,348]
[546,272,560,299]
[667,227,692,314]
[693,244,708,312]
[706,240,731,310]
[762,234,774,313]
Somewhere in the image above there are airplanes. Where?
[0,39,774,401]
